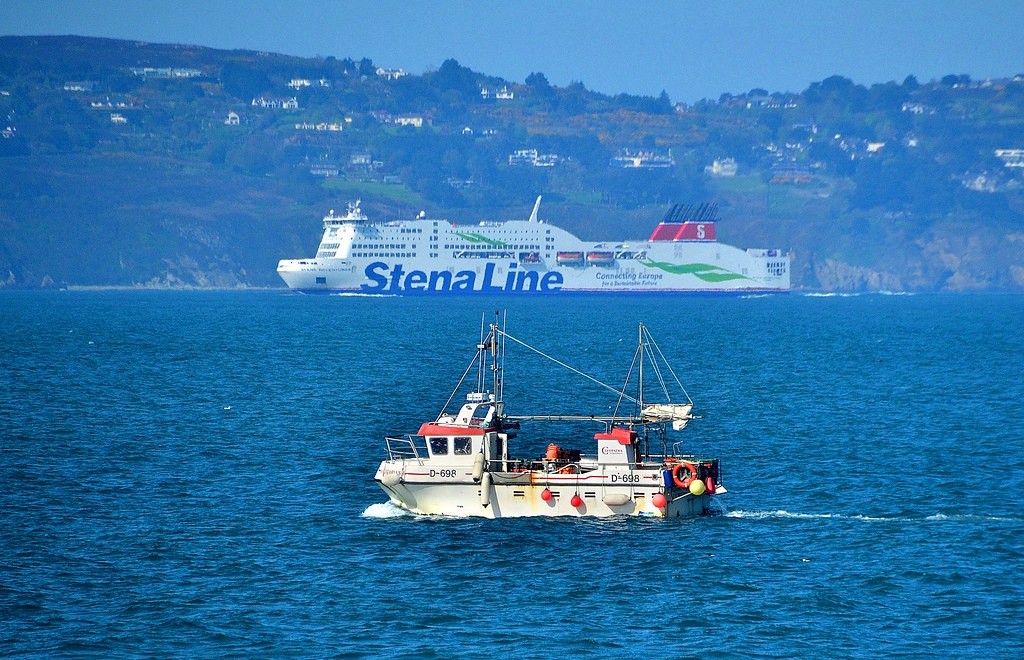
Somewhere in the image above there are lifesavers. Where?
[673,462,697,488]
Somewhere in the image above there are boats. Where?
[524,254,539,263]
[556,251,582,264]
[586,251,614,264]
[373,307,728,520]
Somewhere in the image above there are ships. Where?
[276,194,798,298]
